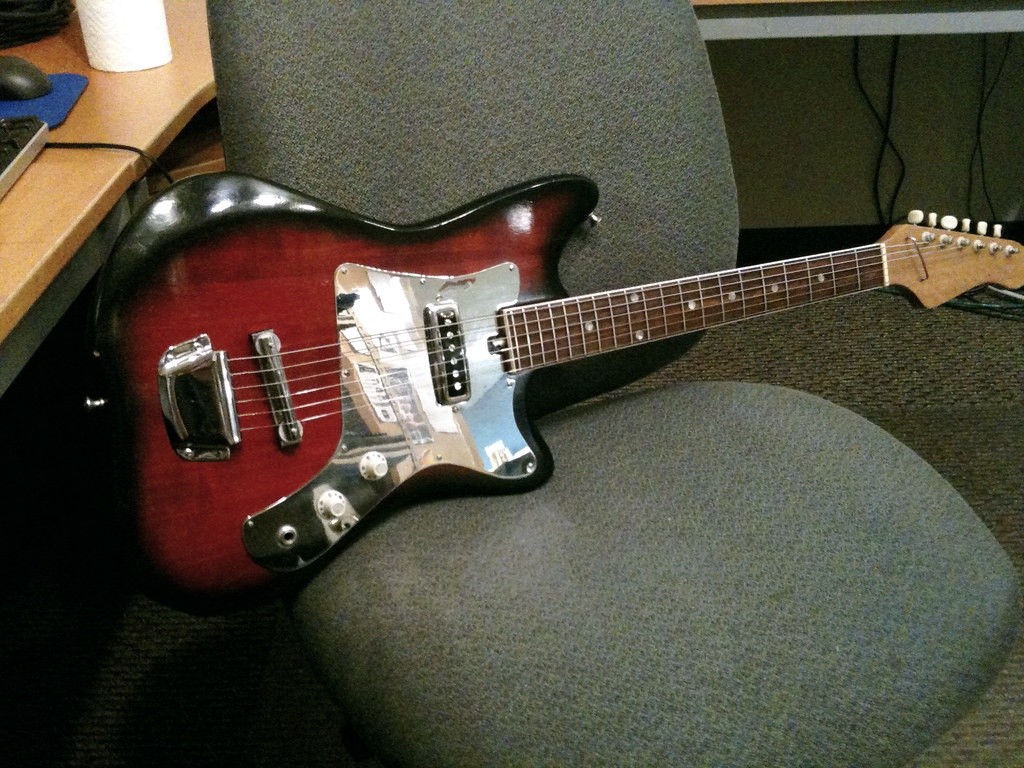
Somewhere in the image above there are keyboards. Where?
[0,115,49,201]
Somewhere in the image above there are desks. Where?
[0,0,218,344]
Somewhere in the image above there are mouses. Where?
[0,55,53,101]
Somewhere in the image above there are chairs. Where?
[204,0,1024,768]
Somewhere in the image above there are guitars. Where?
[84,170,1024,592]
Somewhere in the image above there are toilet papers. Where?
[75,0,173,72]
